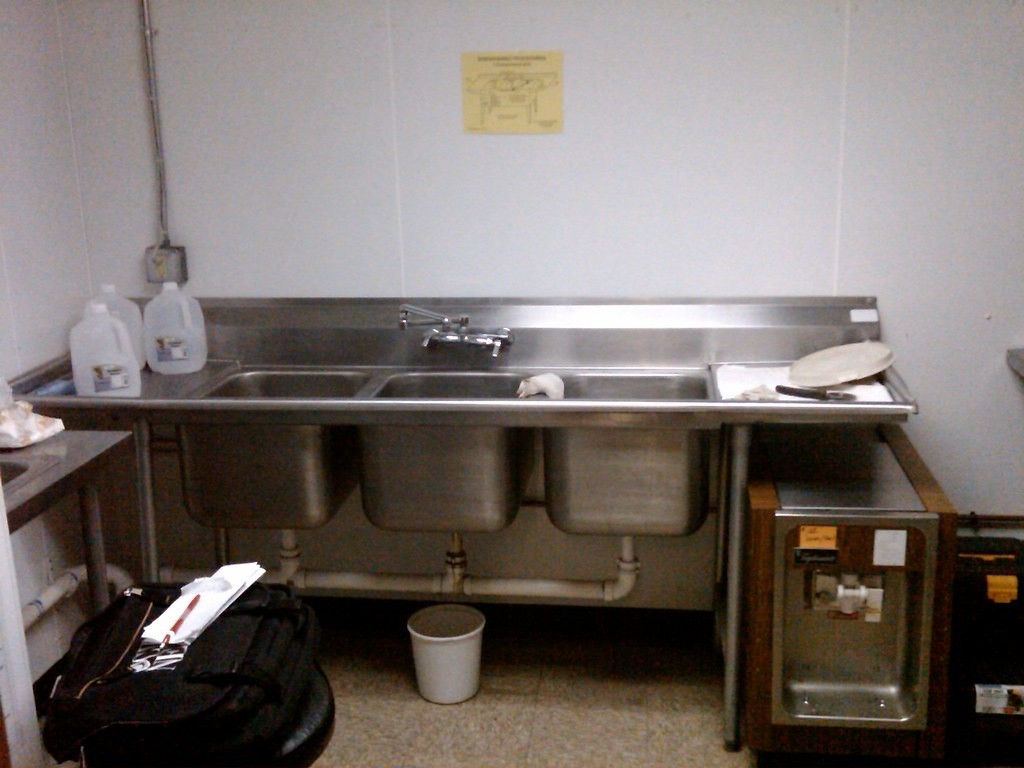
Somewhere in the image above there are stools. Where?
[85,647,336,768]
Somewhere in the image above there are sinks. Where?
[176,369,358,532]
[356,371,531,532]
[538,376,715,538]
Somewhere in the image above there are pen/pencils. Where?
[159,594,201,649]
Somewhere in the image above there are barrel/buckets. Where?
[69,304,142,398]
[406,602,486,705]
[143,280,209,376]
[83,283,146,371]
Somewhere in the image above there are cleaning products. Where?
[69,280,207,398]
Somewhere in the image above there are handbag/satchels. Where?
[31,582,336,768]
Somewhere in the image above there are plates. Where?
[788,341,895,387]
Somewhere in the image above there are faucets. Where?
[396,302,515,359]
